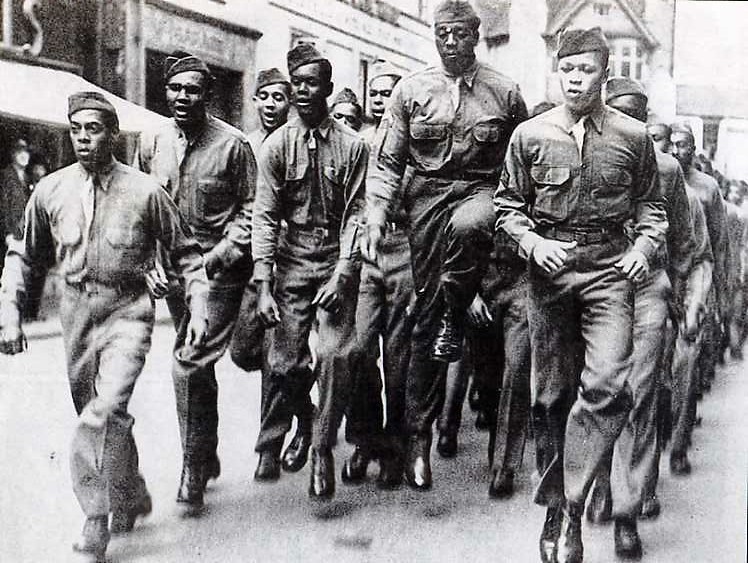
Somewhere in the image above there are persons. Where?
[231,0,748,563]
[0,91,209,561]
[138,51,257,501]
[0,138,54,320]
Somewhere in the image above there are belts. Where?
[69,281,118,293]
[535,226,626,245]
[286,223,340,240]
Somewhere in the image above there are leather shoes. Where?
[429,304,464,362]
[73,382,713,563]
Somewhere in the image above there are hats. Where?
[254,68,291,96]
[433,0,477,26]
[695,153,748,194]
[67,92,119,135]
[165,56,211,89]
[671,123,695,144]
[557,26,609,58]
[605,77,648,106]
[287,44,332,75]
[368,59,402,86]
[8,140,32,155]
[331,87,362,116]
[646,114,670,130]
